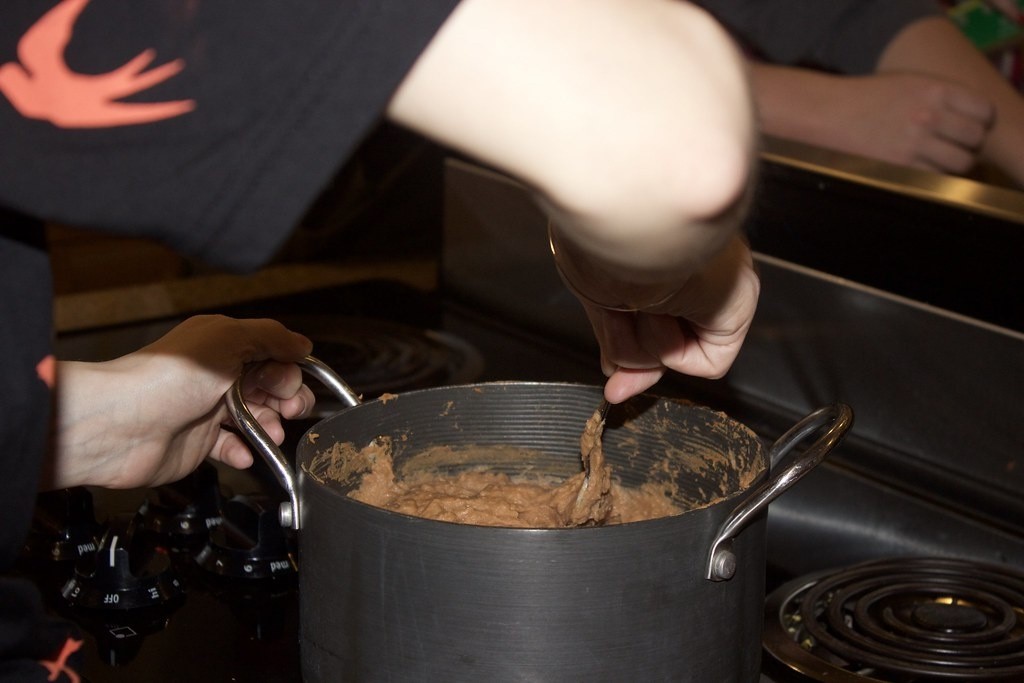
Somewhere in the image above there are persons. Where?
[0,0,760,499]
[696,0,1024,189]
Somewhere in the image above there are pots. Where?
[224,343,856,683]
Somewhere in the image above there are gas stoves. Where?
[56,275,1023,683]
